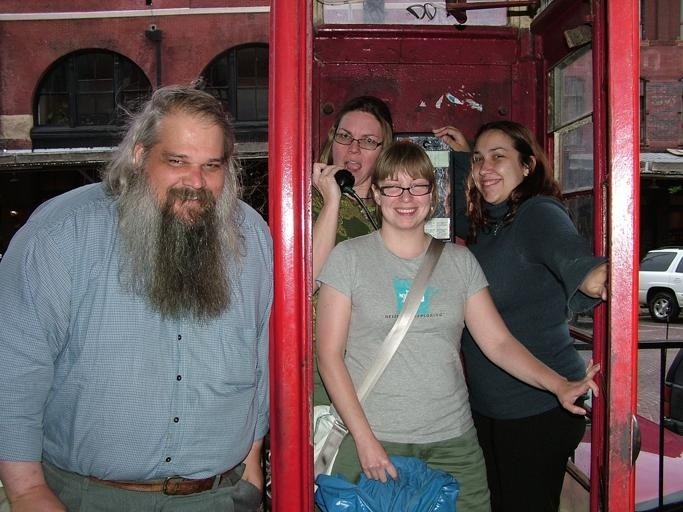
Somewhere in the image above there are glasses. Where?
[334,134,383,150]
[373,182,432,197]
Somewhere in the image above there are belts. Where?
[101,476,221,495]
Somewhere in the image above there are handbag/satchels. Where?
[266,405,349,498]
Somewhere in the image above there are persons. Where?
[310,138,603,511]
[298,93,392,491]
[428,118,620,512]
[0,84,278,508]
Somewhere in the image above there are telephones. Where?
[334,169,353,192]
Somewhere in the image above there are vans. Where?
[637,245,683,322]
